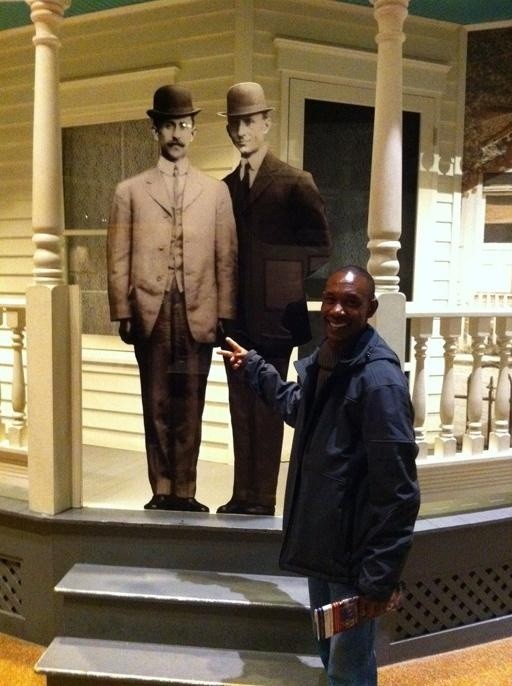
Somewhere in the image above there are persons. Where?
[211,263,422,686]
[214,80,333,518]
[105,83,241,514]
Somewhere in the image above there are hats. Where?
[216,81,275,118]
[146,84,202,119]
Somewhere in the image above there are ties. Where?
[241,164,250,196]
[173,168,180,209]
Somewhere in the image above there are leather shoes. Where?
[145,494,174,510]
[218,500,276,516]
[175,497,209,513]
